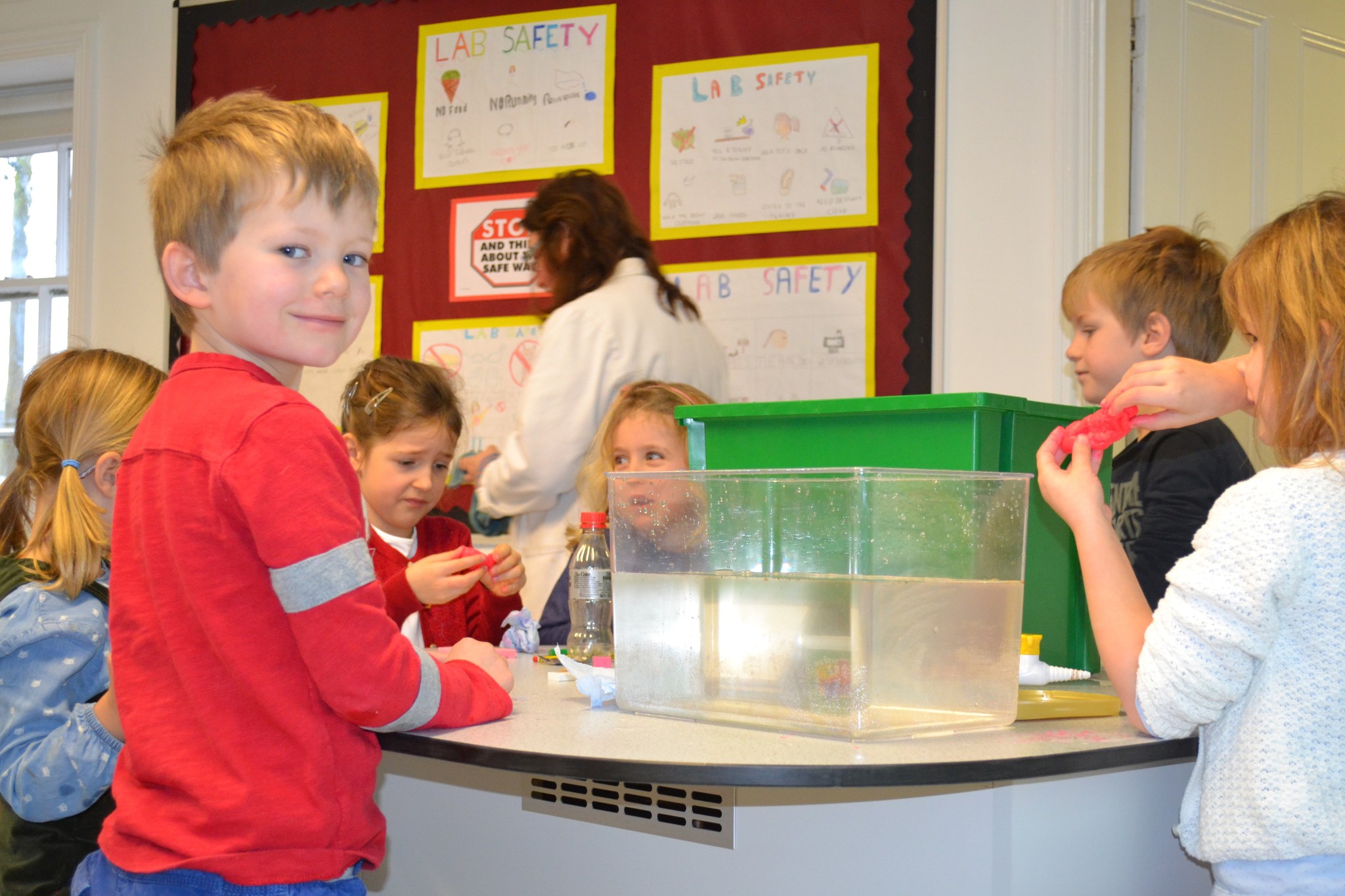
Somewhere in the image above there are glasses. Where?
[523,240,543,265]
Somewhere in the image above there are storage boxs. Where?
[674,392,1113,672]
[603,467,1033,743]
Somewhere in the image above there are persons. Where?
[1,347,723,896]
[1058,224,1261,622]
[457,169,722,650]
[74,97,516,896]
[1036,197,1345,895]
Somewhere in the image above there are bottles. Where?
[567,511,615,667]
